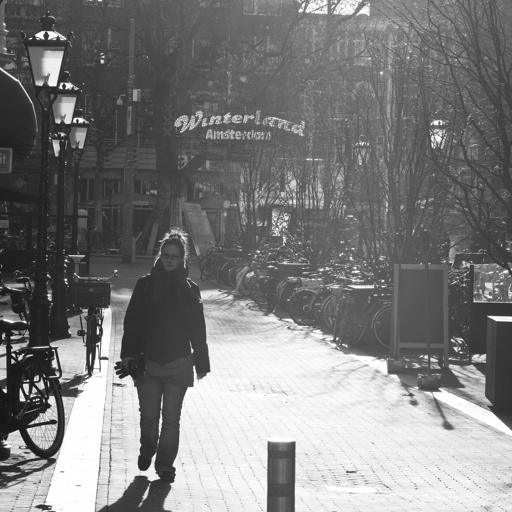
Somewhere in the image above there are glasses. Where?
[159,255,179,260]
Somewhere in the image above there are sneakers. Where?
[138,454,151,469]
[158,471,175,480]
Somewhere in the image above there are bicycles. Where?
[69,270,119,378]
[197,231,472,352]
[0,317,66,459]
[1,229,81,317]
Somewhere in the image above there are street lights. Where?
[48,129,69,283]
[20,10,75,370]
[64,107,91,316]
[49,70,83,340]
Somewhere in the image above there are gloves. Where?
[114,357,148,379]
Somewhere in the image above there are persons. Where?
[120,228,210,482]
